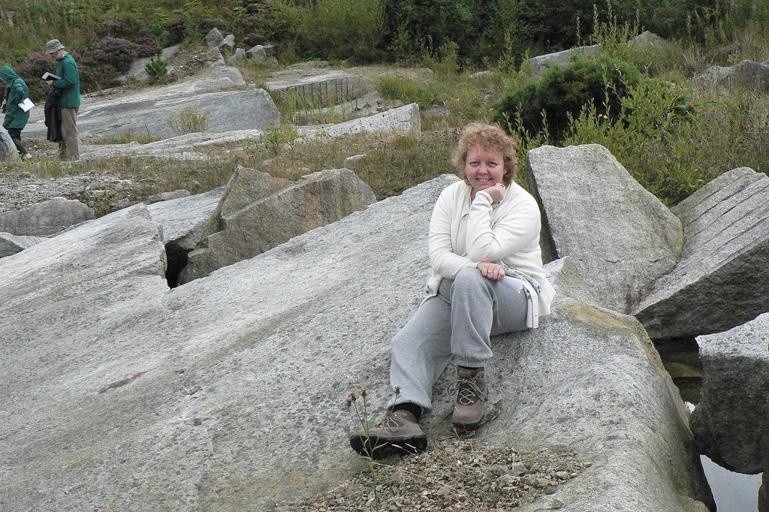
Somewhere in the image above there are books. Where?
[41,71,62,81]
[17,97,35,112]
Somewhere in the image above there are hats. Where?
[46,39,65,53]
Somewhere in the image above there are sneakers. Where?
[447,366,489,428]
[22,153,31,160]
[351,415,427,461]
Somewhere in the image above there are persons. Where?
[349,123,556,460]
[46,38,81,162]
[0,66,32,162]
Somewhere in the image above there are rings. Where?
[488,271,492,273]
